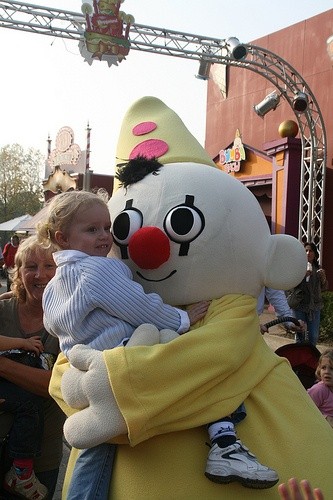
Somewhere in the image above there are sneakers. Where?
[204,438,278,490]
[4,466,49,500]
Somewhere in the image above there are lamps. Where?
[253,90,280,120]
[194,50,212,81]
[292,91,309,114]
[224,36,248,62]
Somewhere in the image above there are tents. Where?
[0,214,39,231]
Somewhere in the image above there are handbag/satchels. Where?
[286,275,310,312]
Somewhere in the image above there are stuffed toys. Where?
[48,96,333,500]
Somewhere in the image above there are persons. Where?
[3,235,21,292]
[36,191,280,500]
[257,285,307,335]
[0,235,66,500]
[0,333,48,500]
[285,242,326,348]
[307,350,333,429]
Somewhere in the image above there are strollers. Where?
[260,317,322,392]
